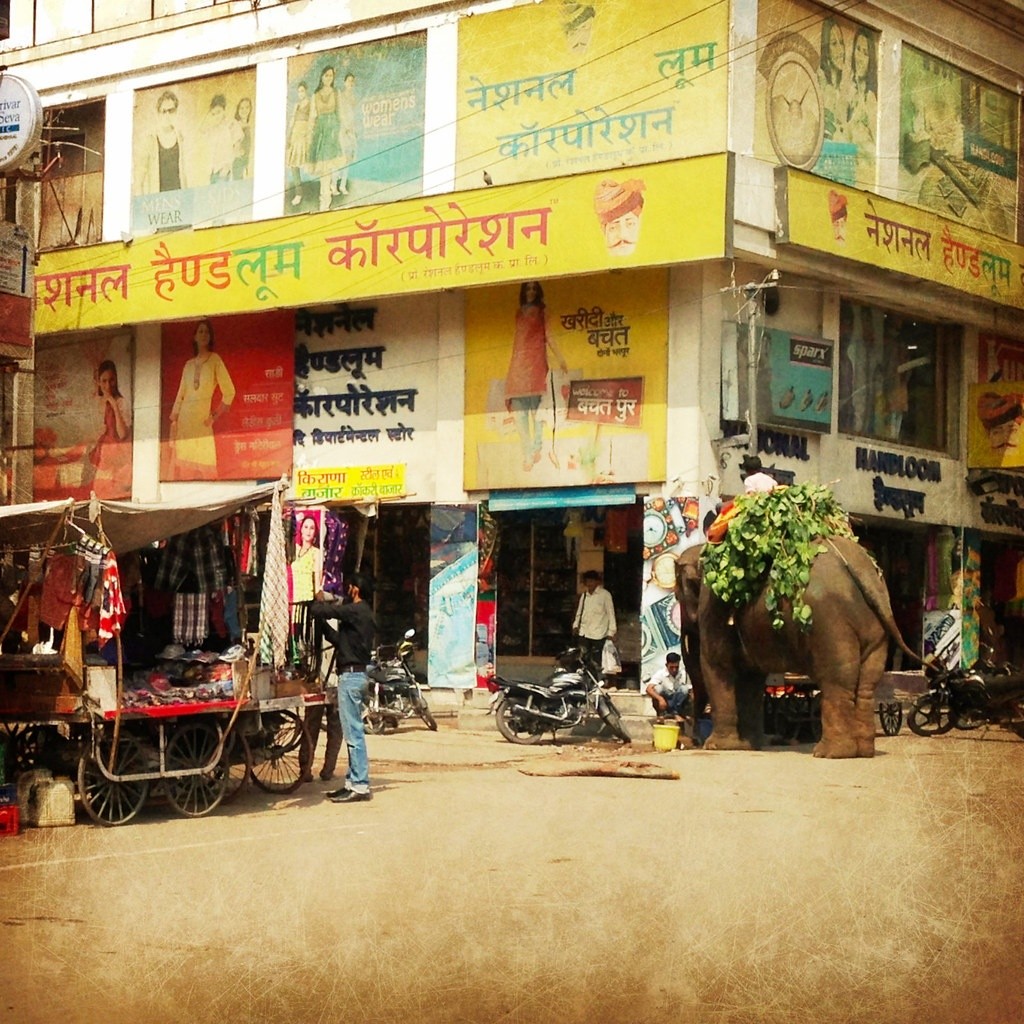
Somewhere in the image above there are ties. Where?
[194,364,201,390]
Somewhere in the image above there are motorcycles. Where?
[907,660,1024,738]
[319,628,438,734]
[486,644,633,744]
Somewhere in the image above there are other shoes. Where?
[673,715,684,722]
[657,715,664,723]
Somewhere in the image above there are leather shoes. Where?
[332,791,370,802]
[327,788,352,799]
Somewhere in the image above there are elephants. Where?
[673,537,940,758]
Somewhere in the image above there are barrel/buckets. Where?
[652,723,680,751]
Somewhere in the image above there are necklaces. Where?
[298,545,312,558]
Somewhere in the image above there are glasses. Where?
[162,108,177,114]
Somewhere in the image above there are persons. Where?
[743,456,778,495]
[506,281,567,471]
[980,579,1011,669]
[310,573,374,803]
[169,319,235,480]
[843,27,876,176]
[289,515,323,604]
[845,307,1024,470]
[886,558,921,673]
[816,17,855,142]
[84,359,131,483]
[596,177,648,257]
[572,570,617,687]
[136,64,355,213]
[646,652,695,724]
[828,193,851,245]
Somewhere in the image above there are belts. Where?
[337,665,366,675]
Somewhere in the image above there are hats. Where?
[218,644,246,662]
[157,645,185,659]
[193,651,218,665]
[175,650,203,663]
[342,574,371,599]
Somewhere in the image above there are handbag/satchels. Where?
[606,640,622,672]
[496,392,516,434]
[487,383,507,429]
[569,629,579,646]
[602,644,616,675]
[550,369,583,433]
[535,368,567,421]
